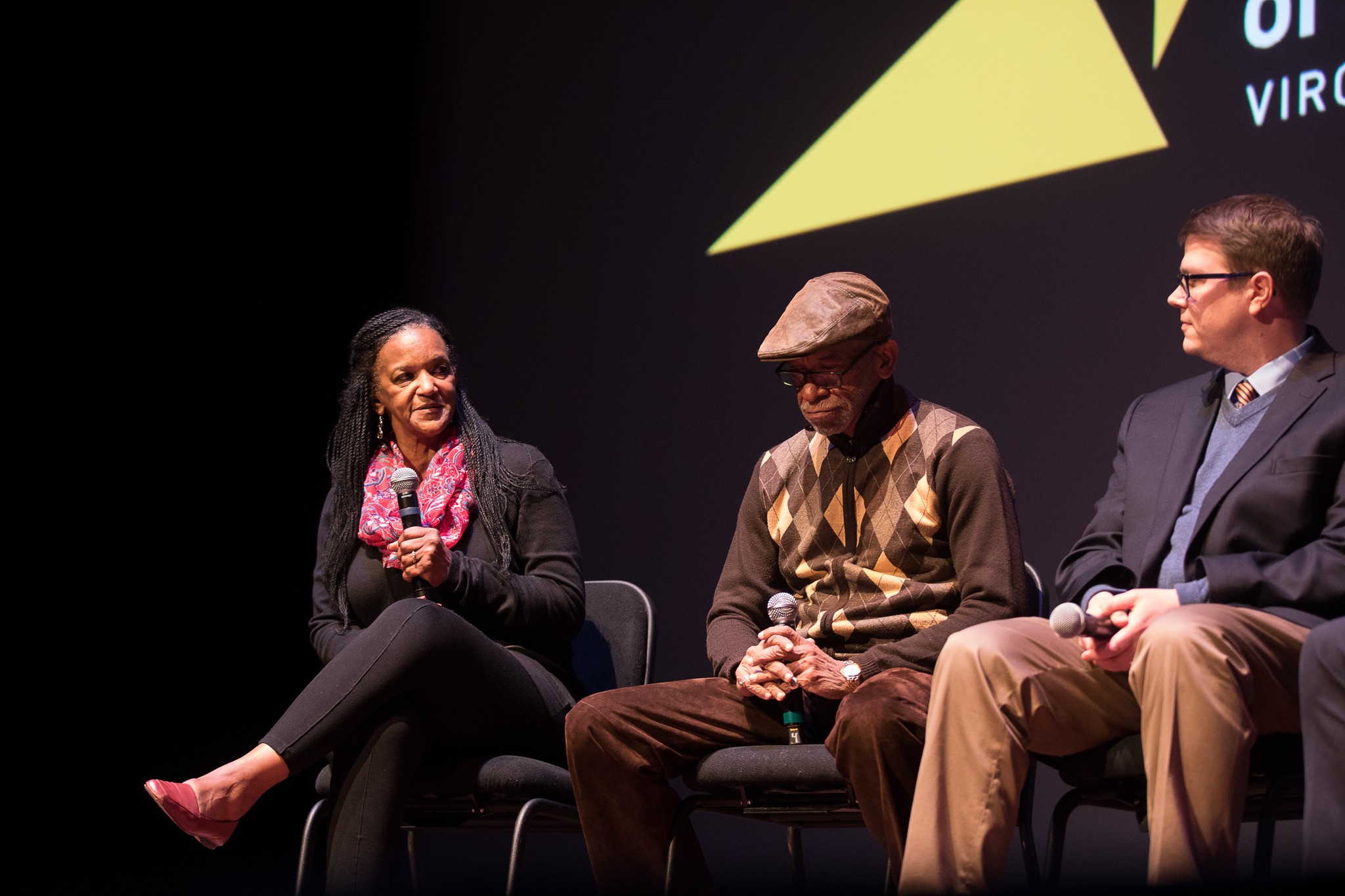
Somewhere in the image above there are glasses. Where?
[774,340,879,390]
[1175,273,1277,300]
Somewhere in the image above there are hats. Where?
[757,272,892,364]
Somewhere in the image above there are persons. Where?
[897,190,1345,896]
[1299,614,1345,876]
[564,272,1026,896]
[143,308,587,896]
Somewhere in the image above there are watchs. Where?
[839,660,861,694]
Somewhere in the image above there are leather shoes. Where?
[144,778,241,851]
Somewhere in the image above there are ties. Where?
[1234,378,1255,409]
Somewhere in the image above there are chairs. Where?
[1017,720,1307,896]
[661,555,1052,896]
[292,573,655,896]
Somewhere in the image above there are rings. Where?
[412,551,416,563]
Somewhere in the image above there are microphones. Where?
[767,592,803,746]
[1049,602,1121,640]
[391,466,433,599]
